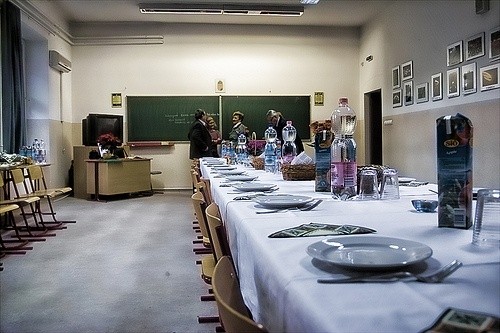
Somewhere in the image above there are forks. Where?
[256,200,323,214]
[316,259,464,283]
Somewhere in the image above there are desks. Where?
[0,154,51,231]
[83,158,154,203]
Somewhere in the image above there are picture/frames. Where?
[391,24,500,109]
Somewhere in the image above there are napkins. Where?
[267,222,377,238]
[219,182,247,187]
[233,193,294,200]
[397,182,430,187]
[417,307,500,333]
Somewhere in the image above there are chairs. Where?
[190,157,270,333]
[0,165,77,271]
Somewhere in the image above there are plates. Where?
[203,157,315,208]
[304,233,432,268]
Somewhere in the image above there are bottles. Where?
[330,97,358,201]
[237,132,248,165]
[264,124,278,174]
[221,139,235,164]
[280,121,298,170]
[31,138,47,164]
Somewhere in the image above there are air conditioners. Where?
[49,50,72,73]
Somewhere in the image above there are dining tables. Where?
[199,156,500,333]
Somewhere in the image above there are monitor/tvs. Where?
[82,114,123,147]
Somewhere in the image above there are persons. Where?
[228,111,250,152]
[266,110,305,156]
[188,109,214,159]
[205,115,221,158]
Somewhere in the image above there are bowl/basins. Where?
[411,199,438,213]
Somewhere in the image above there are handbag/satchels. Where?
[115,148,128,158]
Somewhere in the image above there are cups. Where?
[357,168,380,201]
[472,188,500,248]
[379,169,401,200]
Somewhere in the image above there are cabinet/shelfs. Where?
[73,145,130,201]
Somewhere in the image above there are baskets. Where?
[282,164,315,180]
[357,166,389,193]
[254,158,265,170]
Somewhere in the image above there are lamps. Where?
[139,2,304,16]
